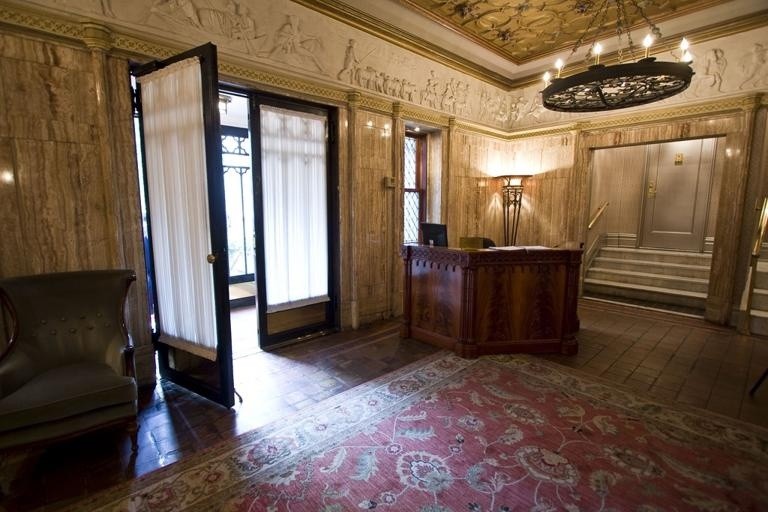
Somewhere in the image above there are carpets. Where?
[39,349,768,512]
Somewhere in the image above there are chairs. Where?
[1,269,144,475]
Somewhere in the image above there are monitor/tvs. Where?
[459,236,484,249]
[420,222,448,247]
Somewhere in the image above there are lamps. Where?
[496,173,533,246]
[540,1,697,113]
